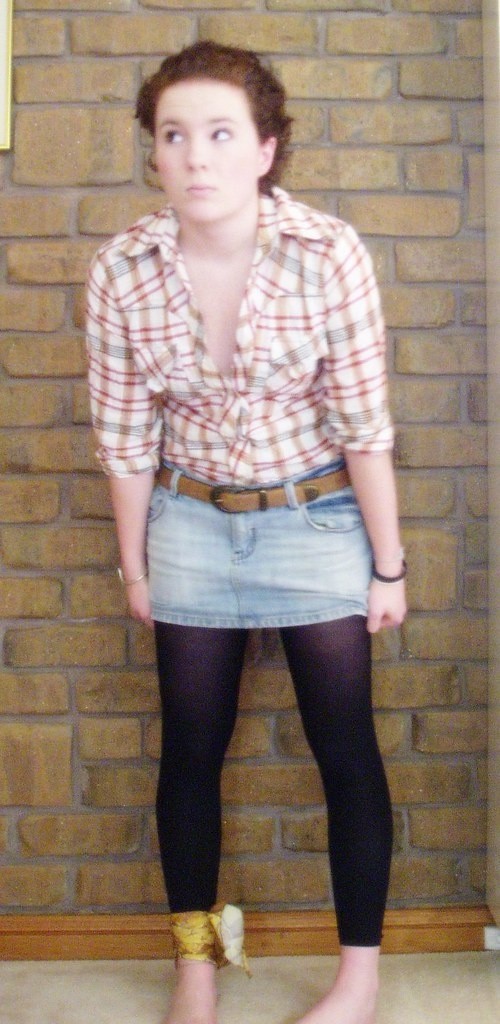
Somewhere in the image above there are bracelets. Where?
[117,568,148,586]
[372,560,407,586]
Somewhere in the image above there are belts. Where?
[158,466,351,513]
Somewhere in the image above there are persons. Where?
[82,41,406,1024]
